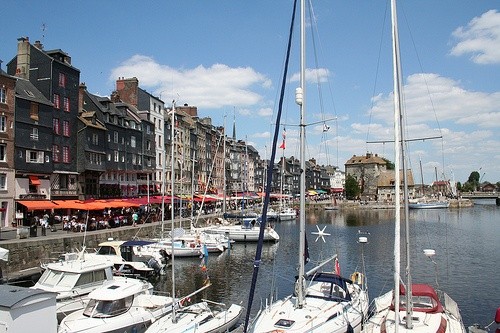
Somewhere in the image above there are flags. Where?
[335,257,340,277]
[280,127,286,150]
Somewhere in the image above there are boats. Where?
[94,240,163,281]
[55,281,181,333]
[26,250,154,323]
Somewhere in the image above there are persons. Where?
[32,192,344,233]
[434,191,461,200]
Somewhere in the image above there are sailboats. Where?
[132,106,298,257]
[142,100,245,333]
[403,158,474,209]
[324,195,339,210]
[355,0,467,333]
[233,0,371,333]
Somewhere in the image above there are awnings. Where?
[0,188,343,211]
